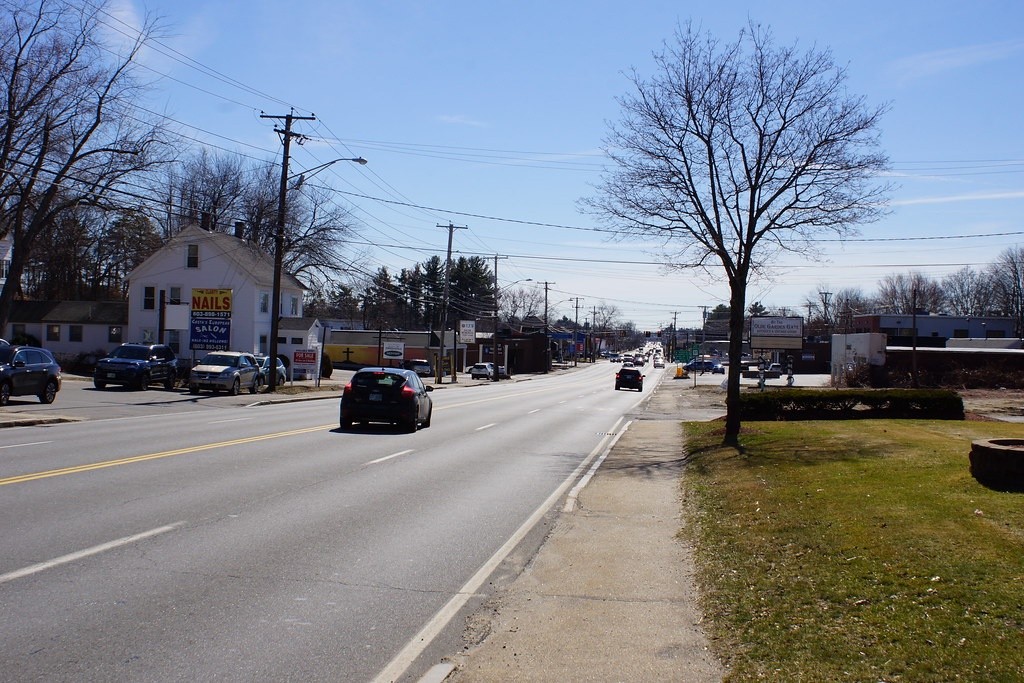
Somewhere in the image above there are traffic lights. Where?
[620,330,626,337]
[646,331,650,338]
[657,331,662,338]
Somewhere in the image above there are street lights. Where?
[544,298,573,371]
[265,157,368,392]
[490,278,533,382]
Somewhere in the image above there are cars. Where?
[0,339,24,362]
[339,367,435,433]
[712,365,725,374]
[465,362,505,376]
[621,342,662,363]
[654,359,665,368]
[257,356,287,386]
[404,359,431,374]
[633,356,645,367]
[471,362,494,381]
[615,368,646,392]
[622,362,634,368]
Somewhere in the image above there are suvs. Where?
[610,353,621,362]
[0,345,63,405]
[768,362,782,375]
[188,350,259,396]
[94,342,178,391]
[683,360,714,372]
